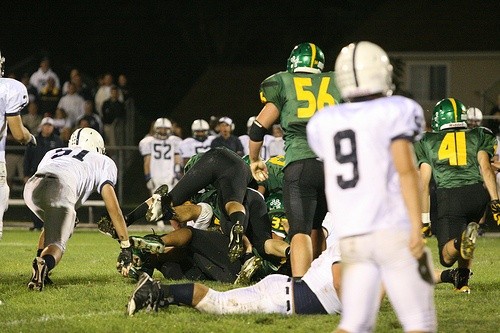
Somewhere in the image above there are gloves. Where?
[491,200,500,225]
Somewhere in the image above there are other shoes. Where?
[453,268,470,294]
[459,222,480,259]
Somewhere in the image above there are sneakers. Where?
[98,219,117,239]
[227,221,243,262]
[129,234,165,253]
[146,184,168,220]
[126,272,156,316]
[233,257,262,284]
[27,258,48,292]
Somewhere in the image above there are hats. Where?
[41,117,54,127]
[218,117,233,125]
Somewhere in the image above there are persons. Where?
[0,42,500,333]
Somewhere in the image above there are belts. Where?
[35,174,58,179]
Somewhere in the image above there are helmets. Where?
[439,97,467,129]
[466,107,483,127]
[247,117,256,127]
[431,99,443,133]
[153,118,171,139]
[287,42,325,73]
[335,40,393,99]
[68,127,104,154]
[192,120,209,141]
